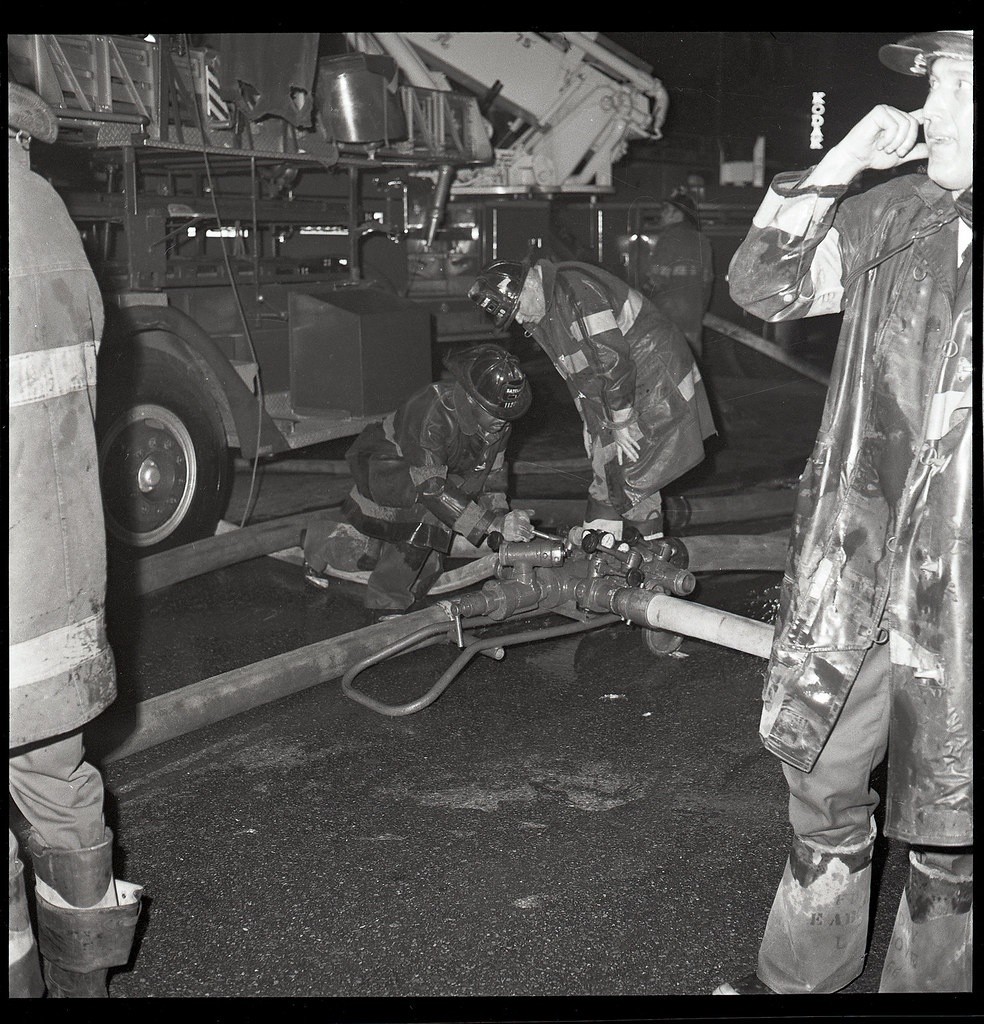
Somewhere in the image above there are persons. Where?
[468,260,717,540]
[712,31,973,995]
[9,80,148,1000]
[342,344,533,621]
[648,192,713,365]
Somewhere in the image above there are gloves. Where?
[485,508,536,542]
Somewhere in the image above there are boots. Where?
[9,858,46,998]
[28,826,118,998]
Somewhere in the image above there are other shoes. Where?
[712,973,772,994]
[300,529,330,592]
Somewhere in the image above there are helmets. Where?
[666,194,702,233]
[466,253,535,335]
[879,29,974,77]
[442,342,532,420]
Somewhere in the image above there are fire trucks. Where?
[9,32,766,567]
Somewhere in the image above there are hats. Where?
[9,81,59,144]
